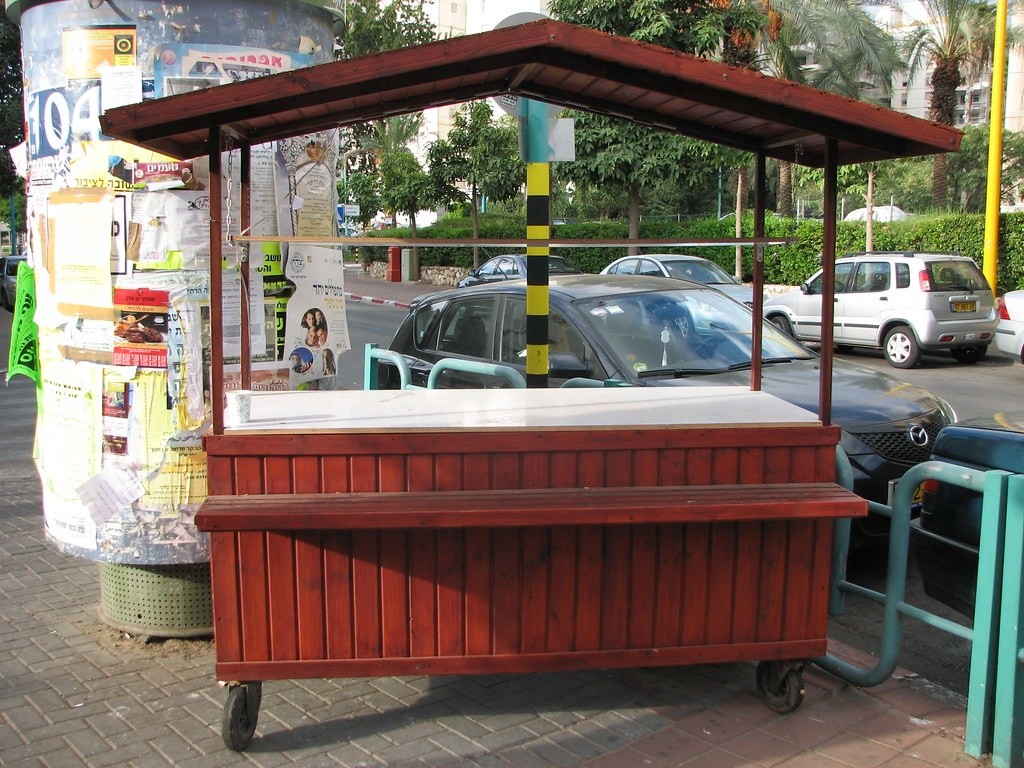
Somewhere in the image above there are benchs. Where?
[441,317,526,358]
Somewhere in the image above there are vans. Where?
[845,205,904,221]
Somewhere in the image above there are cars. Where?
[0,256,27,311]
[916,408,1024,629]
[379,272,961,571]
[995,288,1024,366]
[599,254,771,340]
[553,217,581,224]
[457,253,582,284]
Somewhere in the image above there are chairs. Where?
[862,270,887,292]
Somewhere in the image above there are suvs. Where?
[762,251,1000,370]
